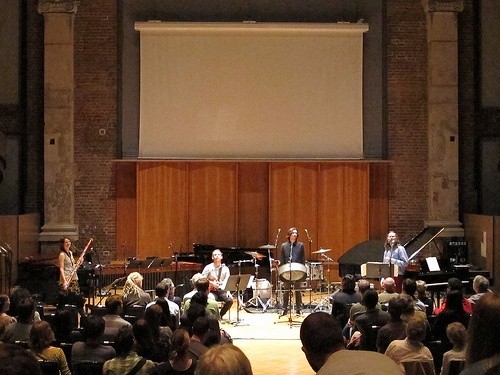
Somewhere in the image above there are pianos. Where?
[337,226,490,312]
[14,251,97,305]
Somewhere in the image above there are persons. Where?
[59,237,85,316]
[0,271,253,375]
[332,274,361,318]
[344,275,500,375]
[383,231,409,293]
[300,312,405,375]
[280,227,304,316]
[202,249,234,323]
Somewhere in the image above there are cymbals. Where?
[245,251,266,257]
[312,248,330,253]
[259,245,276,249]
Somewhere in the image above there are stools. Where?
[96,292,111,305]
[216,301,231,323]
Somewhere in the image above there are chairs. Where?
[344,303,466,375]
[10,302,146,375]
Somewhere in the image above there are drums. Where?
[242,278,272,307]
[277,262,307,283]
[304,261,323,281]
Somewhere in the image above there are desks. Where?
[425,281,469,308]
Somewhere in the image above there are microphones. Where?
[168,243,172,248]
[391,240,395,244]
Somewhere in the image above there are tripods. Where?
[227,232,333,329]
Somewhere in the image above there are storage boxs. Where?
[360,262,394,278]
